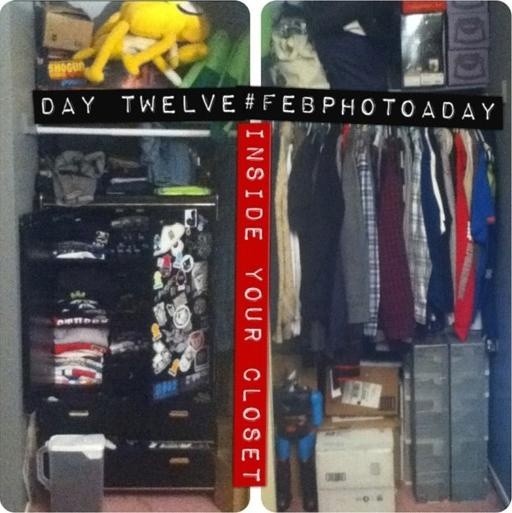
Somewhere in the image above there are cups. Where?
[35,433,111,511]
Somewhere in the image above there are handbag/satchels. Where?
[270,27,330,90]
[309,13,387,91]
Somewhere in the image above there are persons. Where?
[273,368,323,512]
[67,0,215,85]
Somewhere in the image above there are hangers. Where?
[299,119,492,164]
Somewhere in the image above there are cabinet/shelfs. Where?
[315,428,396,512]
[33,195,219,492]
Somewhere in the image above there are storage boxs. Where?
[43,2,96,49]
